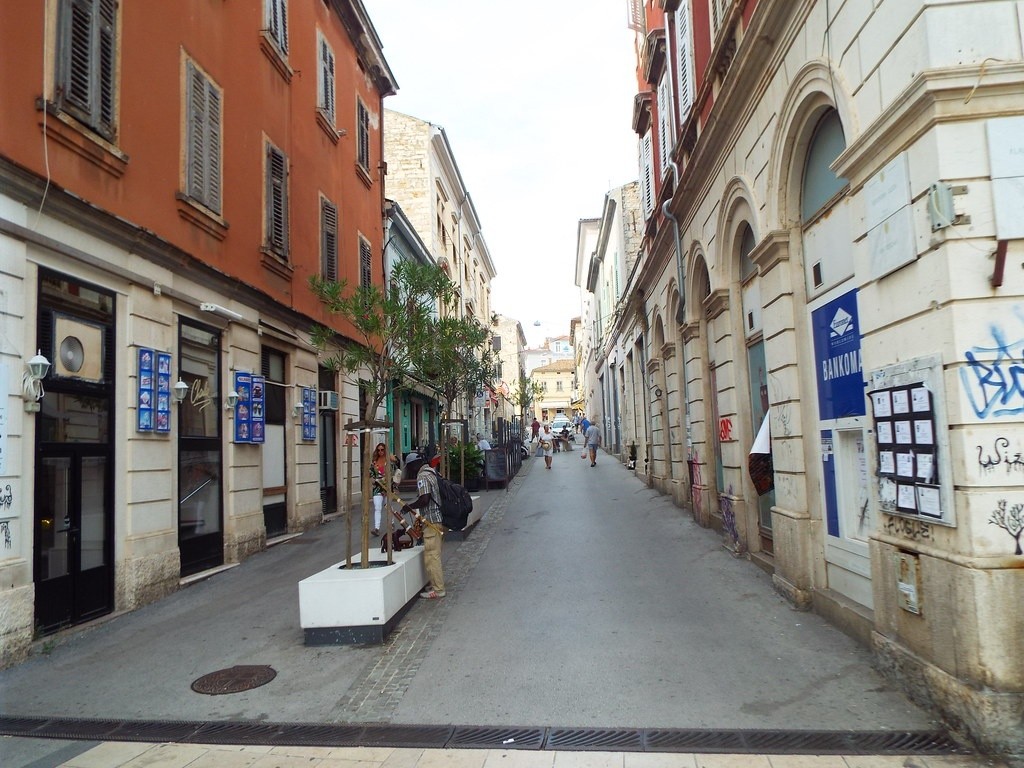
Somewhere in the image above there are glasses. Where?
[377,448,384,452]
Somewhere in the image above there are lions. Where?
[381,529,406,553]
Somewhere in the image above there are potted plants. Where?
[448,439,485,491]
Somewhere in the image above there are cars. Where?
[550,413,575,441]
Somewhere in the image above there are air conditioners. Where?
[318,391,340,411]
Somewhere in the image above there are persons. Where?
[477,433,491,453]
[371,443,400,536]
[400,453,445,599]
[529,412,601,469]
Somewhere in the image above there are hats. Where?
[542,442,551,451]
[402,452,422,469]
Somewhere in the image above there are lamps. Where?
[199,302,242,321]
[172,377,189,404]
[225,389,240,411]
[404,392,414,406]
[436,404,444,416]
[426,401,435,414]
[26,349,51,387]
[292,399,305,418]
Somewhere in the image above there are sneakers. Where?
[420,585,445,598]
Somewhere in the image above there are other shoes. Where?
[591,463,595,467]
[371,528,380,536]
[392,524,396,531]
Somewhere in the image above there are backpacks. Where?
[421,467,473,531]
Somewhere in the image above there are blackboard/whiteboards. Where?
[485,449,508,481]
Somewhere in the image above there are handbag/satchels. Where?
[534,442,543,457]
